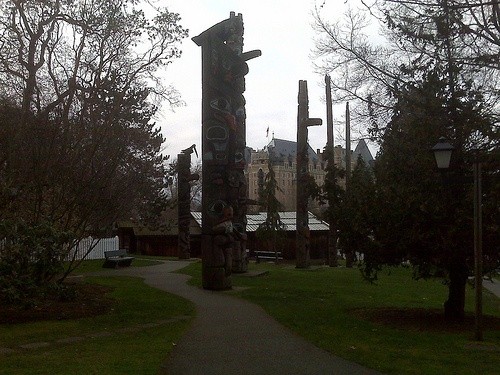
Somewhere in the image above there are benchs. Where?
[254,251,284,264]
[103,249,134,268]
